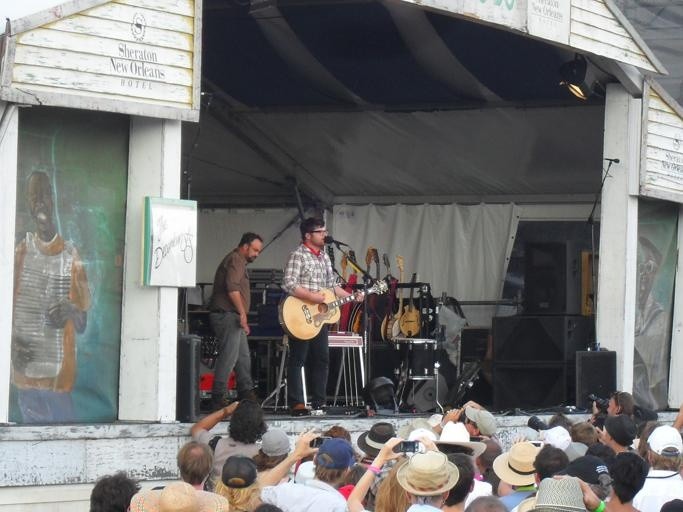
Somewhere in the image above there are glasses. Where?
[310,229,329,235]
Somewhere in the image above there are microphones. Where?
[606,158,619,163]
[324,236,347,246]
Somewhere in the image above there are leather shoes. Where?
[290,403,308,415]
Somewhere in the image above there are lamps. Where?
[555,61,597,104]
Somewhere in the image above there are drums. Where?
[396,338,438,381]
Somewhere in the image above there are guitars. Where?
[278,280,388,341]
[336,249,421,344]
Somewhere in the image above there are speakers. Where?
[524,239,582,316]
[492,316,595,364]
[406,373,449,413]
[576,350,616,412]
[177,334,202,423]
[492,364,576,409]
[459,328,493,411]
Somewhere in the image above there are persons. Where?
[8,168,91,425]
[207,232,263,407]
[89,391,682,512]
[279,218,367,414]
[635,236,671,411]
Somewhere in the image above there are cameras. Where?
[313,437,332,449]
[400,441,426,454]
[588,393,609,413]
[527,416,548,432]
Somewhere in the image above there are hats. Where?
[222,454,257,488]
[316,438,356,468]
[261,428,290,457]
[396,449,460,497]
[646,425,683,457]
[129,481,229,512]
[605,414,638,446]
[492,426,613,512]
[357,404,497,457]
